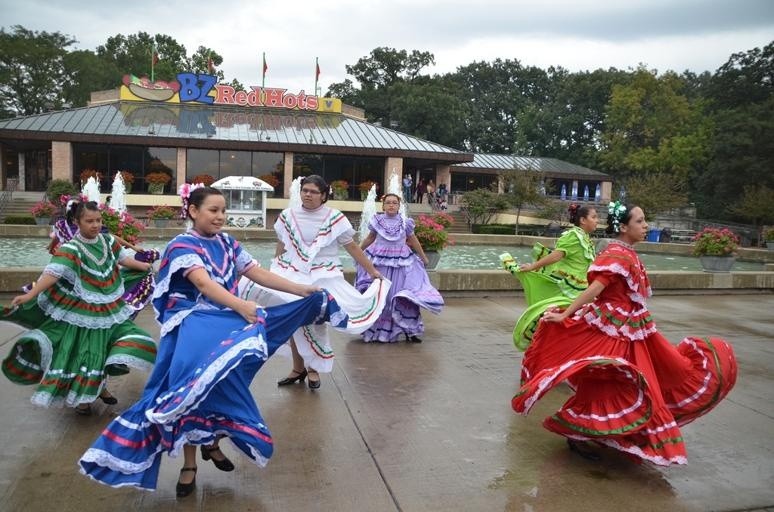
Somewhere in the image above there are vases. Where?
[35,216,51,224]
[420,248,441,270]
[699,253,736,274]
[361,192,368,201]
[333,191,349,201]
[152,216,170,228]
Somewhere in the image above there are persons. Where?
[403,173,450,209]
[269,175,393,388]
[352,193,445,343]
[76,182,349,497]
[499,203,598,353]
[22,193,162,320]
[511,202,738,472]
[0,200,158,416]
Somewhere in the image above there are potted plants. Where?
[113,171,135,193]
[146,172,171,194]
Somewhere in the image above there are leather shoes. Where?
[74,405,91,416]
[568,438,599,461]
[98,395,117,405]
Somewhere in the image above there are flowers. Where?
[692,226,740,259]
[331,180,351,197]
[31,201,56,217]
[411,212,455,252]
[58,194,146,245]
[359,180,380,193]
[149,204,176,221]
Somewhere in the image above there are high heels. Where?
[176,466,197,496]
[201,444,234,471]
[406,333,422,342]
[278,368,307,385]
[307,371,320,388]
